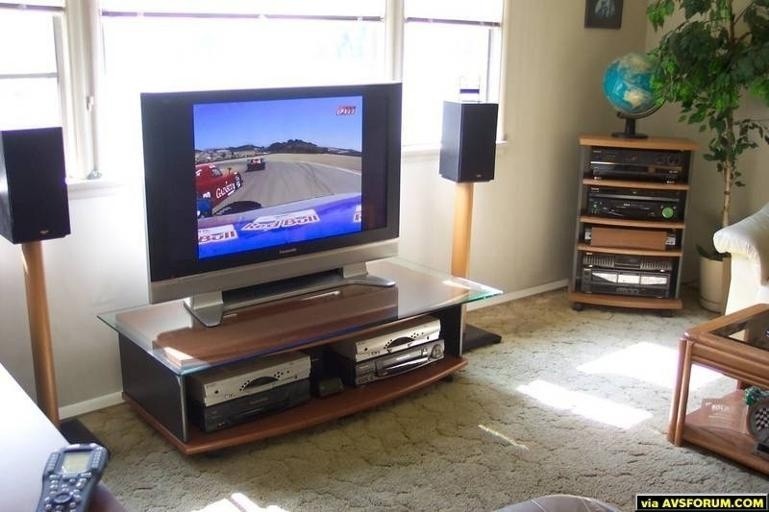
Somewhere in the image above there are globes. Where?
[602,53,673,141]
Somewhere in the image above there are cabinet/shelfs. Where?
[567,130,699,316]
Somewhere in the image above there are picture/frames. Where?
[584,1,624,29]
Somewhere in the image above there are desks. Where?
[94,256,505,455]
[666,303,768,476]
[1,364,130,512]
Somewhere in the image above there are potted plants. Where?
[639,0,769,317]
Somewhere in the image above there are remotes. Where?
[33,441,110,512]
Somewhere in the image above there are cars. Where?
[247,157,265,171]
[195,163,243,218]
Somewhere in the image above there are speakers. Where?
[0,126,72,245]
[438,98,499,184]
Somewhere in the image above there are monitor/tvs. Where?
[140,80,403,329]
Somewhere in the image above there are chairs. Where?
[711,202,768,341]
